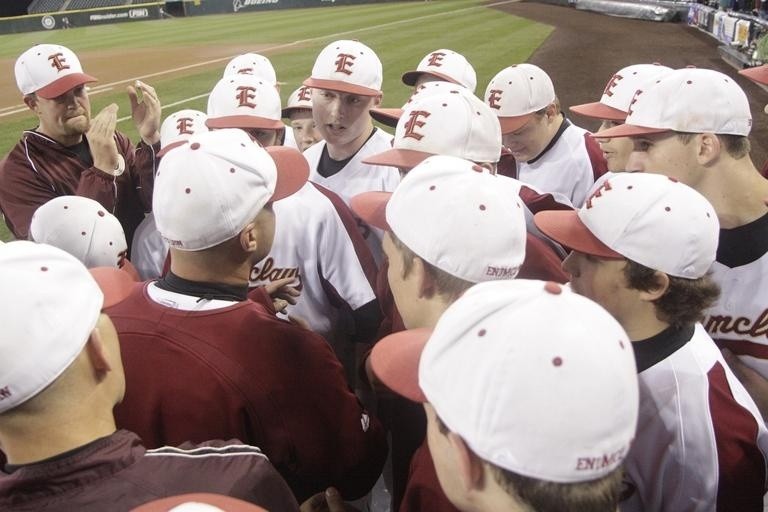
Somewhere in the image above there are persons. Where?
[749,9,760,40]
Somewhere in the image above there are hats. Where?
[566,59,673,119]
[152,127,311,253]
[30,194,139,284]
[588,67,753,140]
[370,280,638,486]
[13,44,96,100]
[2,236,135,412]
[535,167,723,280]
[152,40,558,163]
[348,154,529,278]
